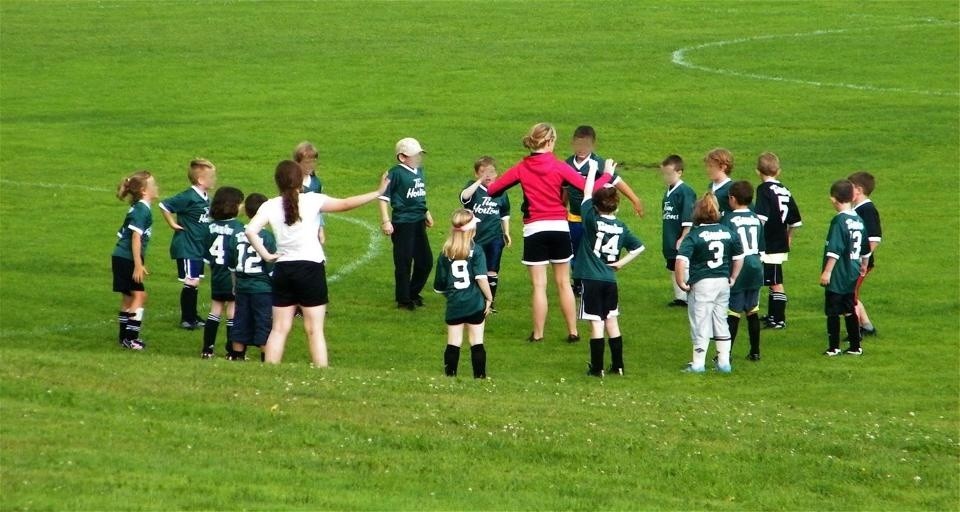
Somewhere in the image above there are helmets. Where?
[395,137,427,157]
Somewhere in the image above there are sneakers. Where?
[823,347,841,357]
[843,348,863,355]
[679,362,705,373]
[767,321,786,329]
[842,335,862,343]
[746,353,760,361]
[667,299,687,308]
[179,321,194,330]
[225,353,251,360]
[527,331,544,342]
[712,355,731,373]
[859,326,876,336]
[567,334,580,343]
[195,318,205,327]
[412,297,423,306]
[713,355,733,363]
[586,367,605,378]
[607,367,624,375]
[396,302,415,311]
[573,284,581,296]
[200,352,214,359]
[759,314,774,322]
[121,339,144,349]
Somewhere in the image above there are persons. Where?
[706,145,736,217]
[245,159,390,368]
[676,192,745,373]
[434,210,492,380]
[487,123,617,343]
[561,125,644,296]
[754,152,804,331]
[378,137,434,311]
[158,159,217,329]
[820,180,871,356]
[293,141,326,244]
[229,193,276,362]
[660,154,697,307]
[711,180,766,364]
[111,170,159,348]
[844,173,876,343]
[202,188,244,360]
[572,158,645,378]
[459,156,511,314]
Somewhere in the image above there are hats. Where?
[452,209,482,232]
